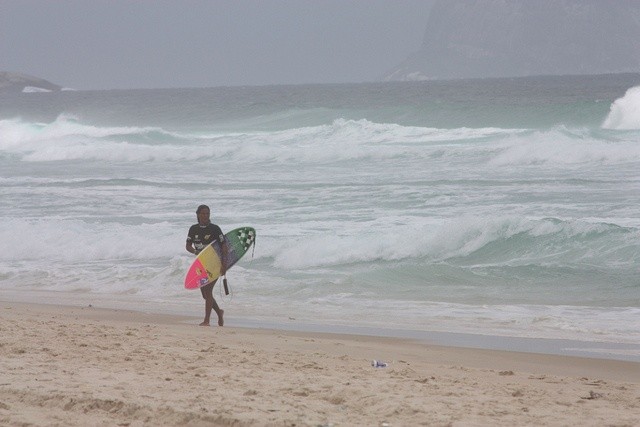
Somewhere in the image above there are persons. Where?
[186,205,228,326]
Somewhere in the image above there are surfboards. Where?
[184,227,256,303]
